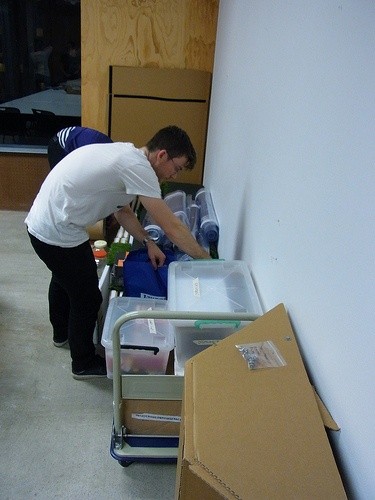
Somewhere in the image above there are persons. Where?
[47,127,166,270]
[22,124,214,380]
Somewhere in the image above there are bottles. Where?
[92,239,107,280]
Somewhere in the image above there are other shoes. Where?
[72,354,107,379]
[53,328,68,346]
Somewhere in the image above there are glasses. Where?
[159,147,184,173]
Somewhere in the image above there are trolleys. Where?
[109,310,266,467]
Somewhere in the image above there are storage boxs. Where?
[103,297,173,381]
[166,257,262,375]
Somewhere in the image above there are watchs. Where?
[142,235,154,250]
